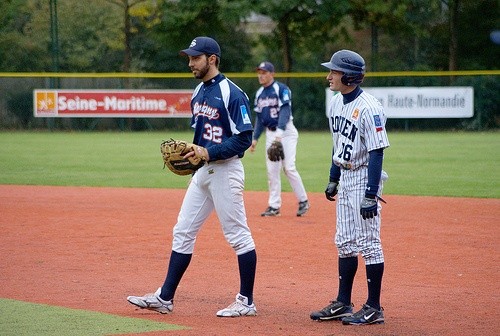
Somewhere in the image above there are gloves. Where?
[324,182,339,201]
[359,194,377,220]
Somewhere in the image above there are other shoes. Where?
[260,206,281,216]
[296,199,310,216]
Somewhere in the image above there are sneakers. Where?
[127,287,174,314]
[216,293,259,317]
[340,303,385,326]
[310,300,355,321]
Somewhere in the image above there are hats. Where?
[321,50,366,75]
[180,37,222,58]
[257,62,275,72]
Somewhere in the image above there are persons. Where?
[126,37,258,318]
[309,49,390,327]
[250,61,310,217]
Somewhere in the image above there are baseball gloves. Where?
[160,141,209,177]
[266,141,284,162]
